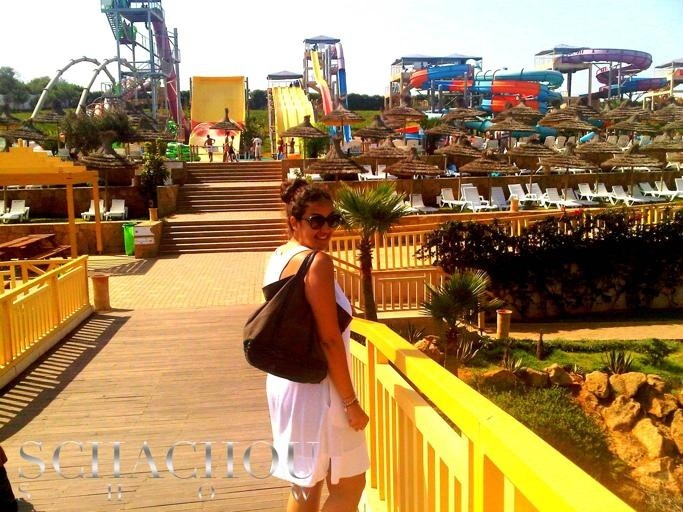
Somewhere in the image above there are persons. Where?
[260,178,372,512]
[252,135,263,161]
[203,134,216,162]
[276,136,285,161]
[224,135,240,163]
[222,131,231,163]
[289,137,295,154]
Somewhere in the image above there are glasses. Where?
[300,213,341,228]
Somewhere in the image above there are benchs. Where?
[0,245,71,274]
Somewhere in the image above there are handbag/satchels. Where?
[244,251,353,384]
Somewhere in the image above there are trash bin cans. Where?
[122,223,137,256]
[92,275,112,311]
[510,199,519,212]
[495,308,513,339]
[149,208,158,221]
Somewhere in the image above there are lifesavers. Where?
[163,142,201,162]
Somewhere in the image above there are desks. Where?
[0,233,56,277]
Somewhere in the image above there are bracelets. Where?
[343,397,358,408]
[342,391,356,403]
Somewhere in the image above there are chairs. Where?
[535,135,683,174]
[81,199,128,221]
[358,165,398,181]
[387,177,683,215]
[287,165,323,181]
[0,200,30,223]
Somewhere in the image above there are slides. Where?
[335,42,352,142]
[561,46,668,99]
[150,7,189,142]
[394,63,564,138]
[309,50,336,139]
[271,84,316,159]
